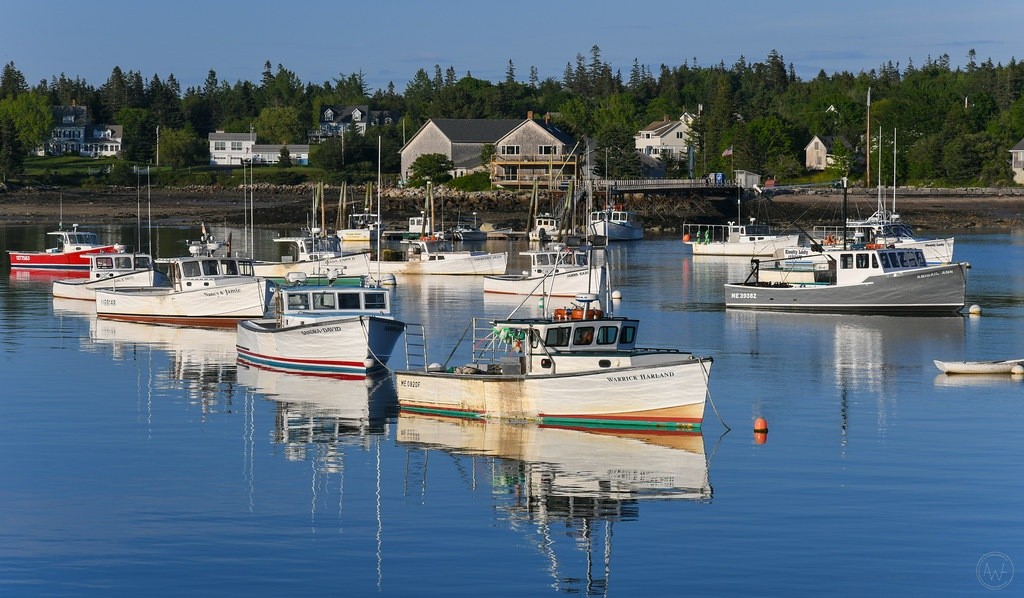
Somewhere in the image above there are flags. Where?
[722,147,732,157]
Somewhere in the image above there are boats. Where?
[235,133,408,376]
[933,373,1024,387]
[934,359,1024,374]
[4,116,972,334]
[394,243,718,432]
[232,356,397,588]
[392,405,714,598]
